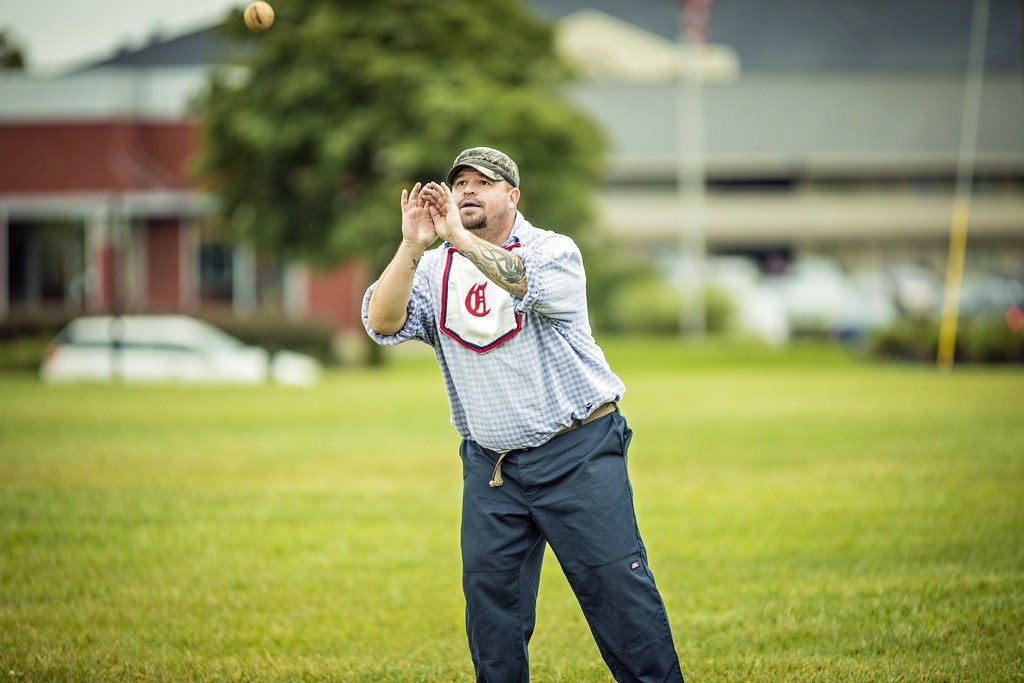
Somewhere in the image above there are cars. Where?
[36,310,322,392]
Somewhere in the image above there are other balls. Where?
[243,1,277,30]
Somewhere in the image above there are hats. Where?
[445,146,521,188]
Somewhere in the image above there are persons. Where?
[360,147,683,683]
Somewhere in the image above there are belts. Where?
[487,402,616,490]
